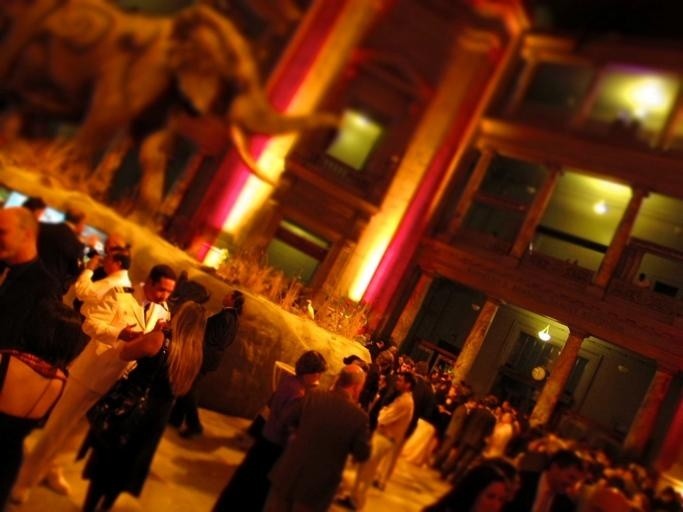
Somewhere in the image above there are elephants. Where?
[1,0,343,232]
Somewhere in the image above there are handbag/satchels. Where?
[87,377,149,448]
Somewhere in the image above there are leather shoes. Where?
[45,466,71,495]
[11,476,31,504]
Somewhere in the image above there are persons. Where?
[0,197,683,512]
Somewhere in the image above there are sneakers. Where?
[334,497,354,510]
[180,428,202,437]
